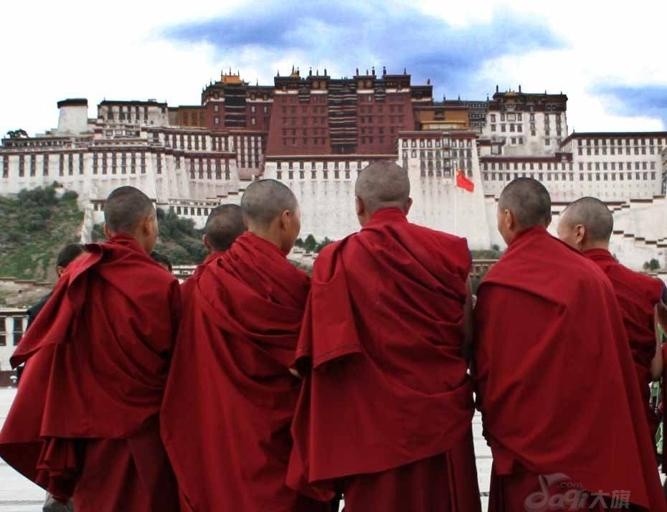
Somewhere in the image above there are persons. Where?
[459,176,666,512]
[144,177,345,511]
[21,242,90,344]
[0,184,186,512]
[282,154,485,510]
[190,202,248,267]
[151,251,174,272]
[548,195,667,468]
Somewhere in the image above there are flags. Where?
[455,172,474,193]
